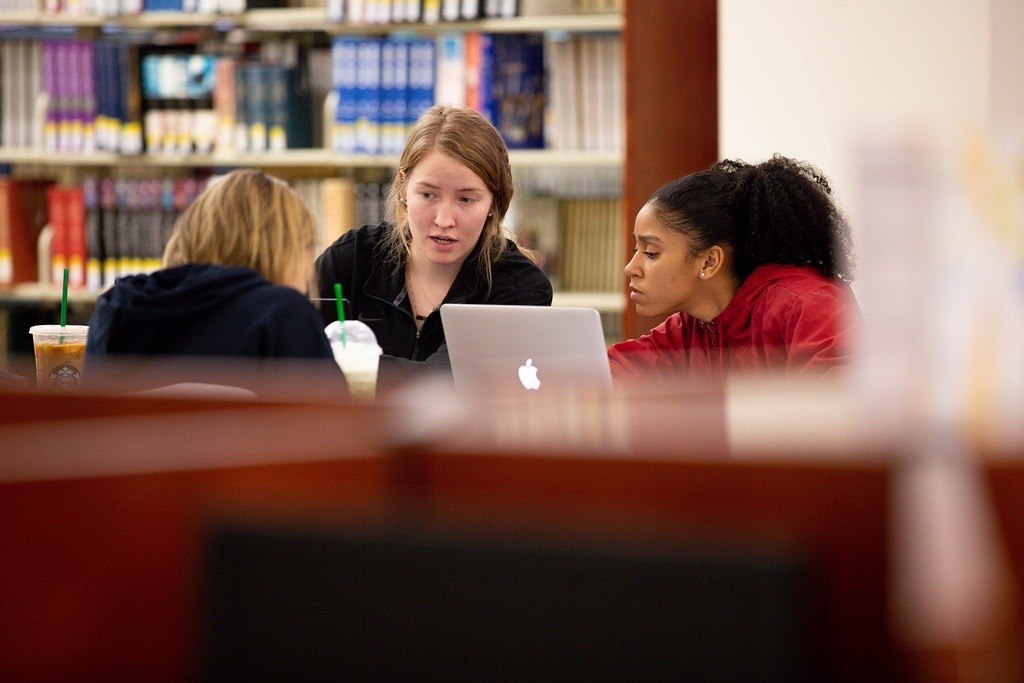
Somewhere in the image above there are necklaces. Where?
[409,263,429,322]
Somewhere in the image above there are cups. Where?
[326,320,383,404]
[29,324,89,393]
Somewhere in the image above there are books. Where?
[2,168,624,299]
[1,0,624,25]
[0,28,624,161]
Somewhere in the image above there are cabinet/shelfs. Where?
[0,0,721,371]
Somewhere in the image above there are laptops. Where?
[440,303,619,415]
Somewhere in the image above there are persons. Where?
[318,105,553,402]
[80,166,337,394]
[606,154,868,403]
